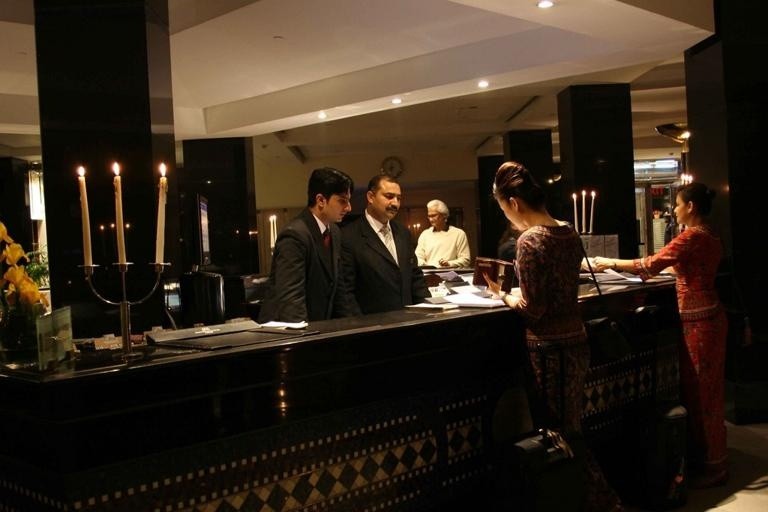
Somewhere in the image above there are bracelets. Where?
[501,292,508,301]
[612,259,616,270]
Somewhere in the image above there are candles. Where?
[78,165,93,264]
[681,174,692,184]
[572,193,579,232]
[112,161,127,261]
[269,215,277,248]
[589,191,595,233]
[581,191,586,232]
[155,157,168,263]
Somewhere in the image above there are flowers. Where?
[0,222,51,314]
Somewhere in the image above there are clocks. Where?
[381,155,403,177]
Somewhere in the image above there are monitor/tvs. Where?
[190,193,212,268]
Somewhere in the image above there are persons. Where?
[484,162,624,431]
[593,183,728,488]
[260,167,470,323]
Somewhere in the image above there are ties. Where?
[323,232,332,256]
[381,225,395,259]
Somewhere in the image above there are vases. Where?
[0,304,37,369]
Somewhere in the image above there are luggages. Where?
[498,342,577,512]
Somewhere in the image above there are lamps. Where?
[655,124,689,143]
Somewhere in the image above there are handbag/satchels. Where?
[585,317,621,358]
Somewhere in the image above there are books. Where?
[404,278,509,312]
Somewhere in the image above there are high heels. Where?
[689,464,729,489]
